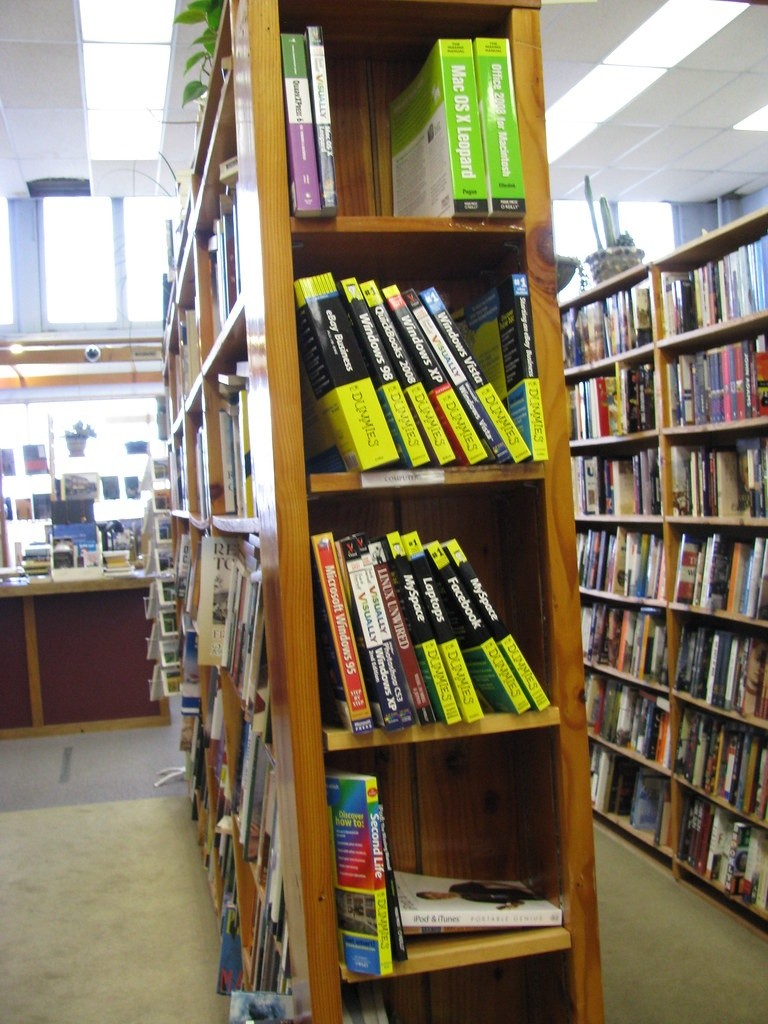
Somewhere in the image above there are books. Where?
[21,542,52,584]
[657,227,768,336]
[583,667,670,851]
[162,57,317,1024]
[559,289,651,370]
[310,531,550,737]
[292,268,550,471]
[570,447,662,516]
[102,548,136,578]
[564,363,655,441]
[324,773,563,1024]
[671,435,768,518]
[673,533,767,718]
[665,334,768,427]
[279,22,339,217]
[388,39,528,222]
[575,526,666,684]
[673,706,768,911]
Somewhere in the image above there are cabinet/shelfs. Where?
[548,206,768,952]
[164,0,607,1024]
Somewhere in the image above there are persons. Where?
[417,879,548,910]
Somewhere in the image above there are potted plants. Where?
[550,174,645,286]
[59,418,98,457]
